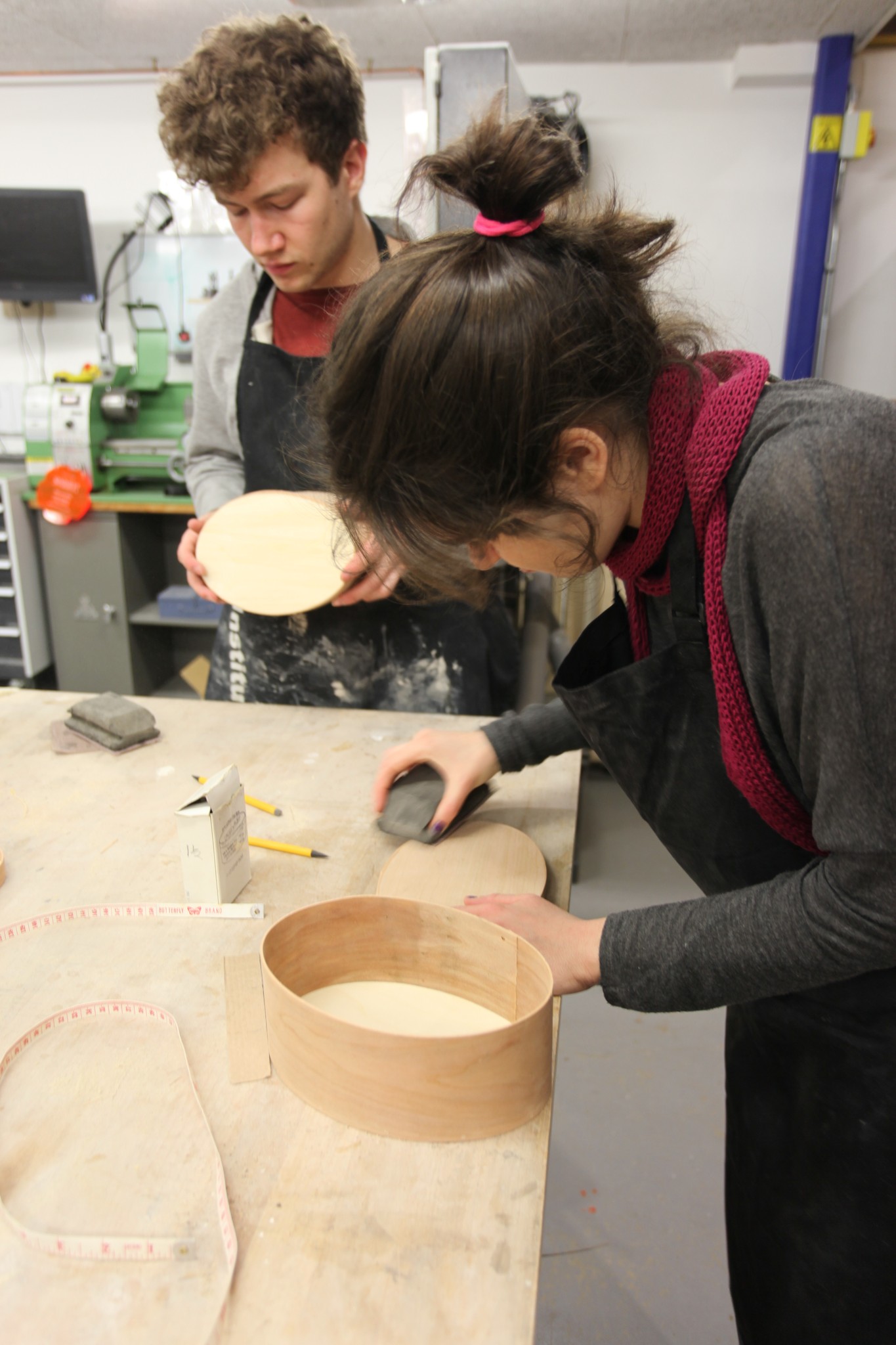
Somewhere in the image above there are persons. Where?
[152,15,527,717]
[286,89,896,1345]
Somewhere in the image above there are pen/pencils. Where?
[192,775,283,817]
[246,834,328,859]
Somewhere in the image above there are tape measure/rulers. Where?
[1,902,263,1345]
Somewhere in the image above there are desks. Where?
[0,684,582,1345]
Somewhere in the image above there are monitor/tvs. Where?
[0,188,102,304]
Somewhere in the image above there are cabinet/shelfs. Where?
[26,495,222,699]
[0,466,54,690]
